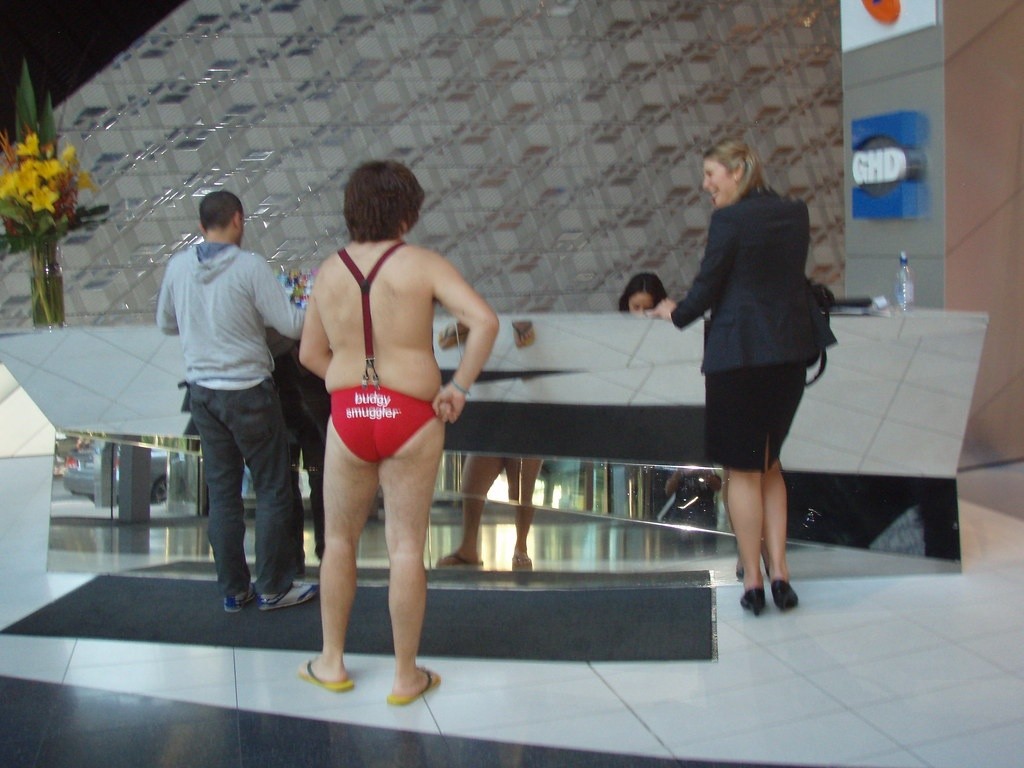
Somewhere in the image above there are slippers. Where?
[386,668,440,706]
[512,552,532,568]
[297,659,353,692]
[436,553,485,569]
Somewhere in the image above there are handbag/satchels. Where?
[805,278,837,382]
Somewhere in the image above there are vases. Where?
[28,237,66,333]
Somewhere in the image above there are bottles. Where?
[895,250,915,314]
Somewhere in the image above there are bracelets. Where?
[451,378,472,397]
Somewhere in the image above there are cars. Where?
[63,442,198,506]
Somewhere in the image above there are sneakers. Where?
[260,582,316,610]
[225,582,257,612]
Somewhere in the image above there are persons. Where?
[618,272,668,314]
[655,464,722,556]
[297,159,499,705]
[271,345,326,579]
[431,453,543,572]
[157,191,320,612]
[650,135,822,614]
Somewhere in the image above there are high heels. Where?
[763,551,769,578]
[736,567,745,581]
[740,587,765,616]
[772,579,798,612]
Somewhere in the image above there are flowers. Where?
[0,53,109,321]
